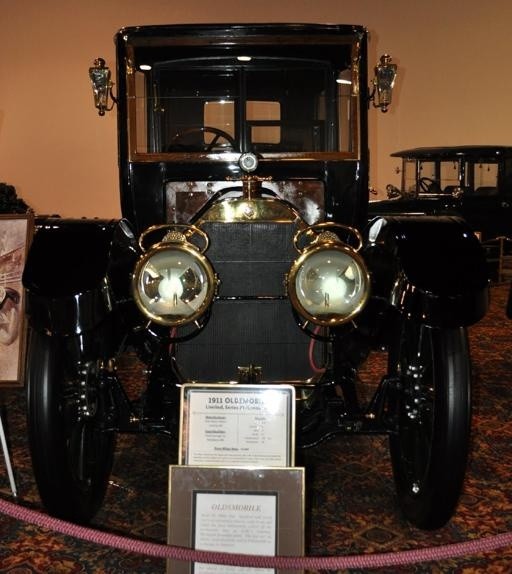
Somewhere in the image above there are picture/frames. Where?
[167,378,308,574]
[0,212,35,390]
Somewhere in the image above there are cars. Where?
[368,146,512,255]
[22,23,491,532]
